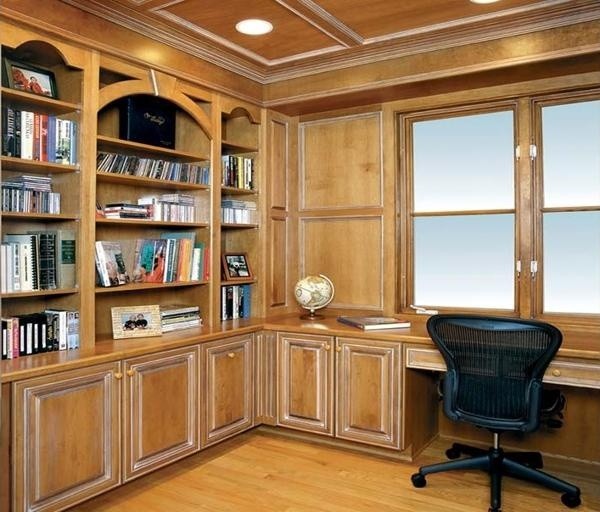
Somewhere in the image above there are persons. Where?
[124,315,136,329]
[136,313,147,328]
[29,77,45,94]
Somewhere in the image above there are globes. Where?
[294,273,334,320]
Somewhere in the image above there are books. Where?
[221,284,251,320]
[1,106,79,363]
[159,302,203,332]
[94,146,205,283]
[221,152,259,226]
[336,314,412,330]
[119,95,176,149]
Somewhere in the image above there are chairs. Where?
[409,313,583,512]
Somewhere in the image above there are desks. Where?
[407,317,600,410]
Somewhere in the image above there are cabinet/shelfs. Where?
[1,40,84,362]
[122,345,199,484]
[216,99,262,322]
[196,331,258,454]
[7,361,122,512]
[92,50,213,344]
[276,332,403,454]
